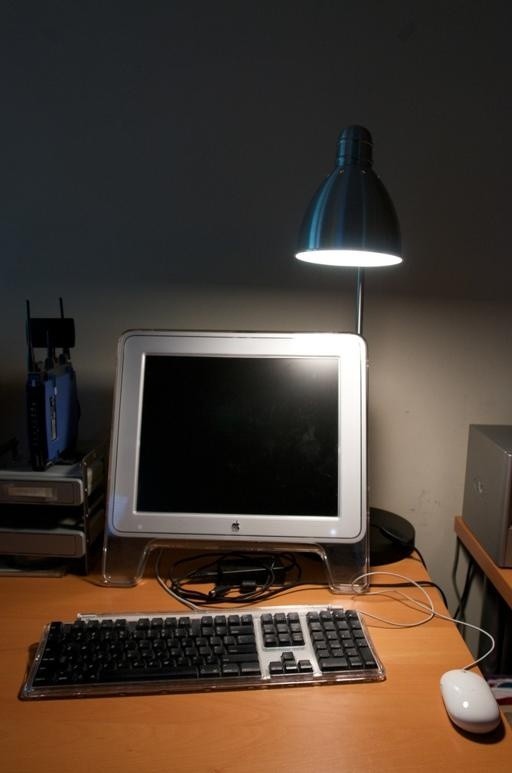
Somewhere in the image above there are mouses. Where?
[438,668,502,735]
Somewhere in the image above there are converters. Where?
[215,558,286,586]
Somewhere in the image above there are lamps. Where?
[293,125,415,566]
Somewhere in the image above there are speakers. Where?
[461,424,512,568]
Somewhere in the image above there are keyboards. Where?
[18,602,387,701]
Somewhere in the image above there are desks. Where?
[0,552,512,773]
[454,516,512,674]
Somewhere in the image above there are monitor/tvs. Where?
[100,326,372,595]
